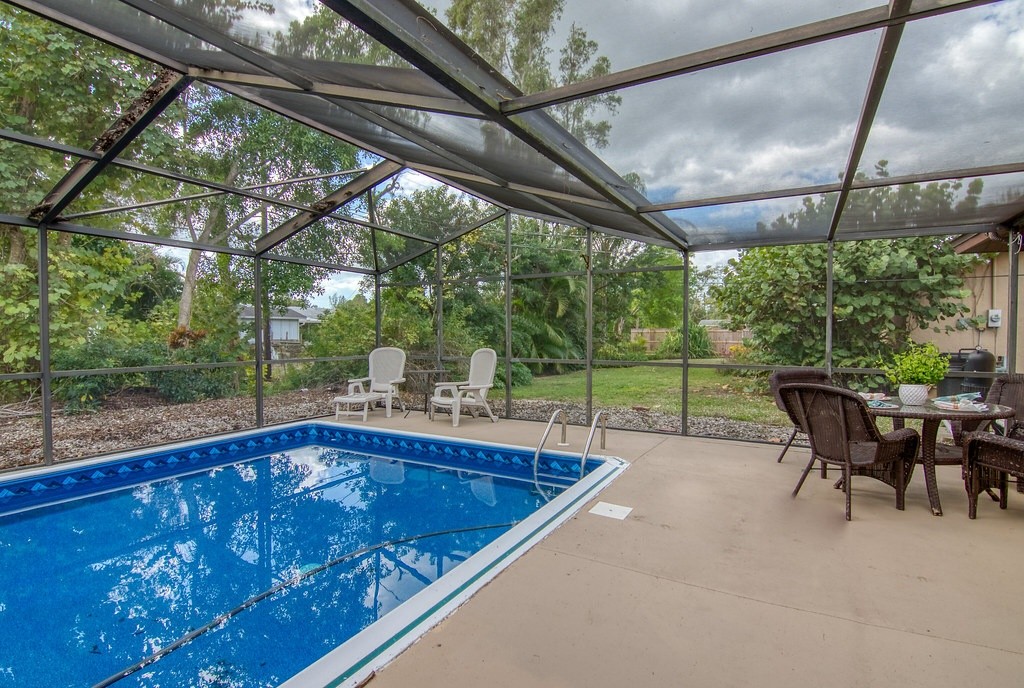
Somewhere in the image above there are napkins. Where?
[931,392,989,413]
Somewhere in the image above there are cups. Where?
[874,392,885,400]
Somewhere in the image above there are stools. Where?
[334,393,382,422]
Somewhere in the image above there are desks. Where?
[404,370,451,418]
[867,398,1016,516]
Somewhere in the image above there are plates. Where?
[869,404,899,409]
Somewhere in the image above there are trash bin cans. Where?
[961,345,996,400]
[937,353,970,398]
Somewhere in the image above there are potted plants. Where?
[875,335,952,405]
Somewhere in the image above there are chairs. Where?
[435,467,497,506]
[348,345,406,418]
[769,368,1024,521]
[370,456,405,484]
[431,348,499,427]
